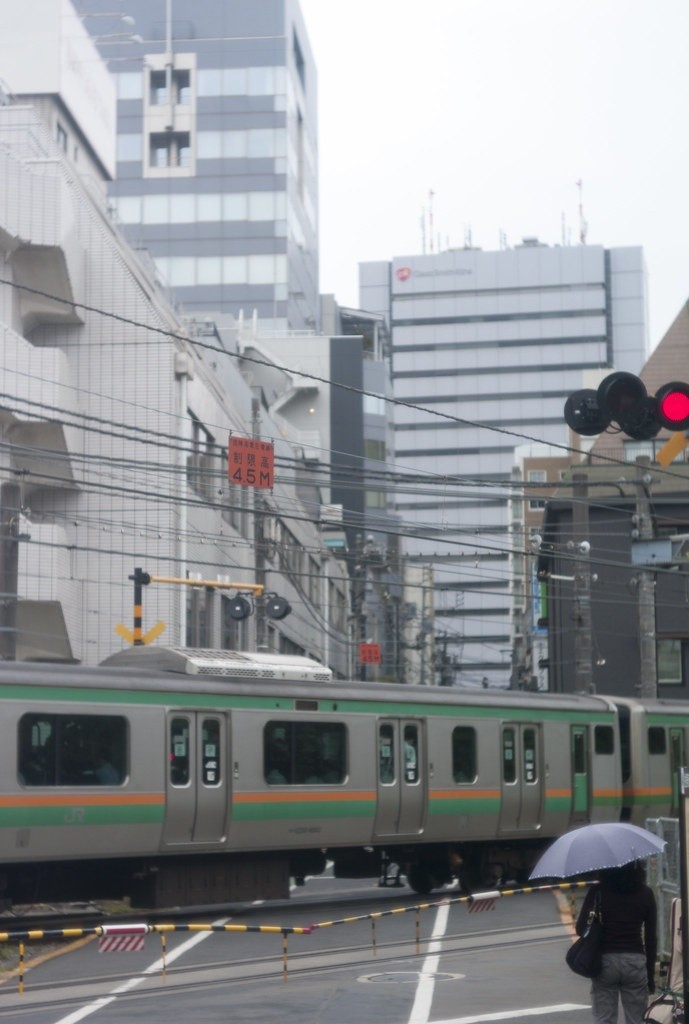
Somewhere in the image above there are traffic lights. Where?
[653,382,689,431]
[597,372,648,422]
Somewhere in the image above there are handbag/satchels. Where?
[566,885,603,978]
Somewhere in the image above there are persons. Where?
[574,848,660,1024]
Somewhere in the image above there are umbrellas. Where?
[528,823,668,883]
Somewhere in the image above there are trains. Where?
[0,645,689,911]
